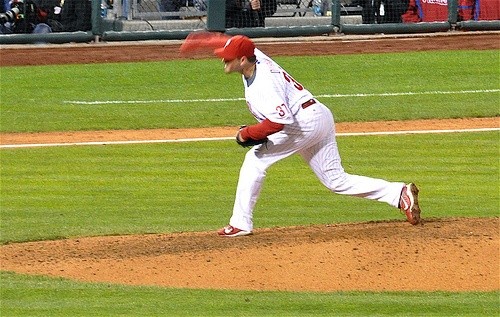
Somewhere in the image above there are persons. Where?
[0,0,93,34]
[361,0,500,24]
[180,31,421,237]
[225,0,277,29]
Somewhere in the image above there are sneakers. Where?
[217,224,253,236]
[399,182,421,226]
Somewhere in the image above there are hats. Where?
[213,35,255,59]
